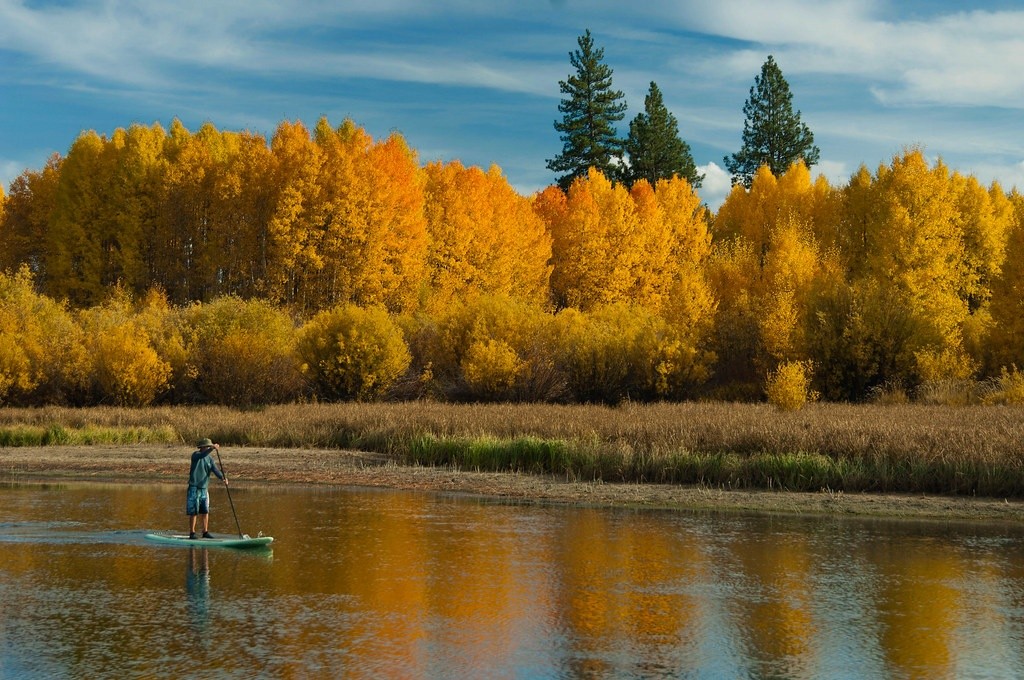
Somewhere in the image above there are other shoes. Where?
[190,532,198,539]
[203,532,215,538]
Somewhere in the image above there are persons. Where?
[186,438,228,540]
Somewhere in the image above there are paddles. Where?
[216,446,242,538]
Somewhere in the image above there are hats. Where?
[196,437,214,448]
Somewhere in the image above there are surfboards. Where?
[146,532,274,547]
